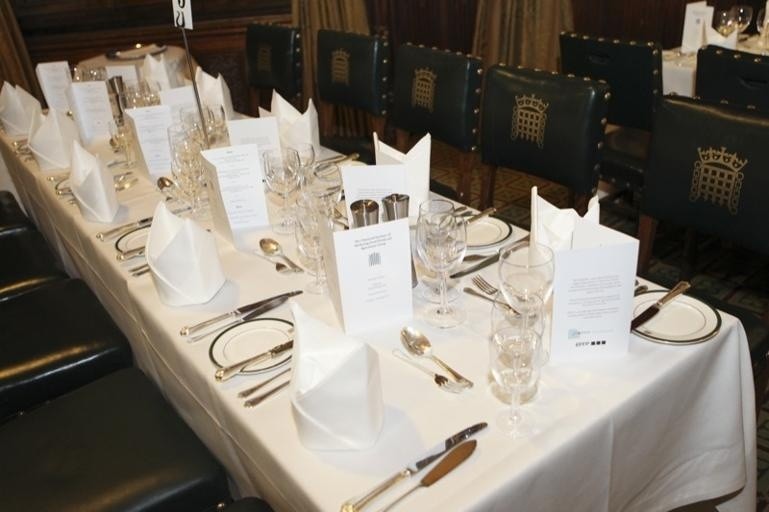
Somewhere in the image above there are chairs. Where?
[389,43,485,203]
[224,495,276,511]
[477,63,613,231]
[0,189,30,232]
[696,44,769,112]
[0,277,131,412]
[558,30,661,223]
[318,26,390,158]
[2,367,223,512]
[637,95,769,365]
[243,21,302,118]
[1,226,65,298]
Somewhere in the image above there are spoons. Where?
[260,238,304,274]
[156,176,175,191]
[68,177,138,209]
[400,326,474,389]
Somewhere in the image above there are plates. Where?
[208,317,301,375]
[629,290,722,346]
[446,214,513,250]
[115,223,164,258]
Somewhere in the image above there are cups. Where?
[200,103,226,147]
[262,148,300,234]
[499,241,555,358]
[416,212,468,330]
[489,290,547,406]
[489,328,540,434]
[167,122,202,200]
[288,143,314,196]
[169,157,205,216]
[295,191,333,234]
[419,199,456,297]
[109,118,137,170]
[295,217,333,294]
[179,104,212,148]
[300,163,342,216]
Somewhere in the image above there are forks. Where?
[255,252,289,273]
[392,349,463,394]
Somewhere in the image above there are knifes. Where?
[178,290,303,336]
[96,215,159,240]
[215,338,293,381]
[630,281,691,332]
[338,421,488,512]
[185,295,288,343]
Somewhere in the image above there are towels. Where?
[142,53,175,89]
[289,299,385,448]
[195,65,234,121]
[27,107,70,172]
[0,81,42,135]
[529,185,600,277]
[374,132,430,216]
[68,138,119,222]
[145,201,227,306]
[258,88,320,158]
[702,20,738,49]
